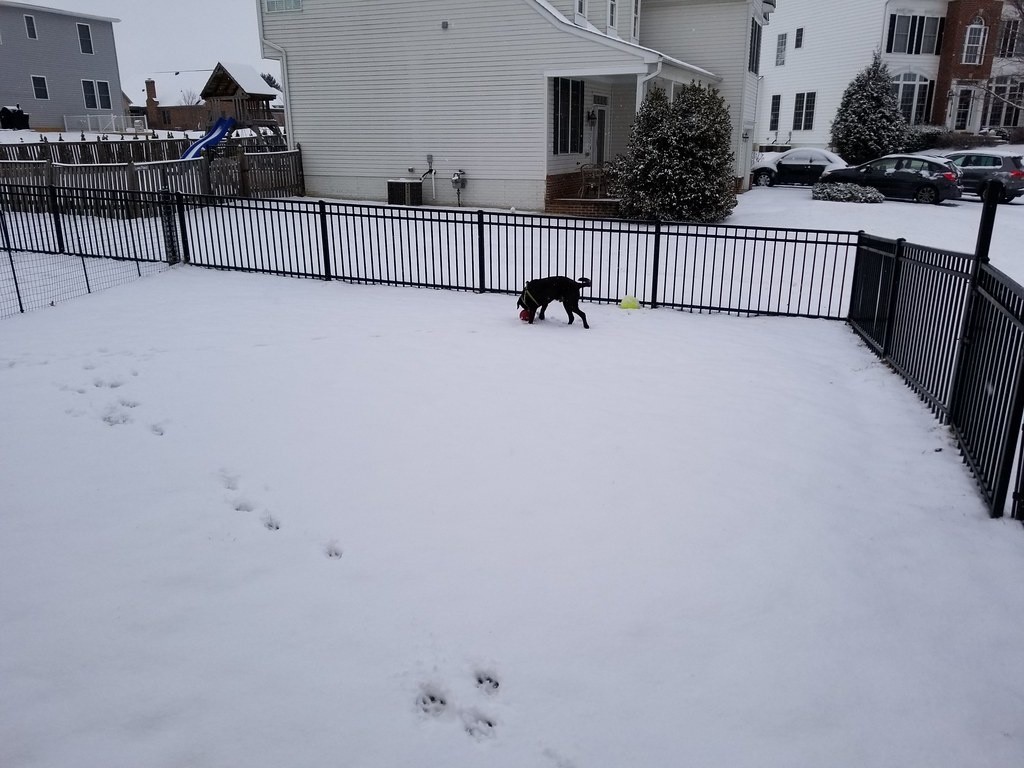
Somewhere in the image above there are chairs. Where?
[581,161,612,196]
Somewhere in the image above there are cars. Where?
[818,154,964,204]
[750,146,849,187]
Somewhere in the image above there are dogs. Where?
[517,276,592,330]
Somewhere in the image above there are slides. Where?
[177,118,236,167]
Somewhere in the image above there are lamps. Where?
[587,110,597,126]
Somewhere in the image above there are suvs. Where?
[944,152,1024,203]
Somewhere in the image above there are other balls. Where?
[520,310,529,321]
